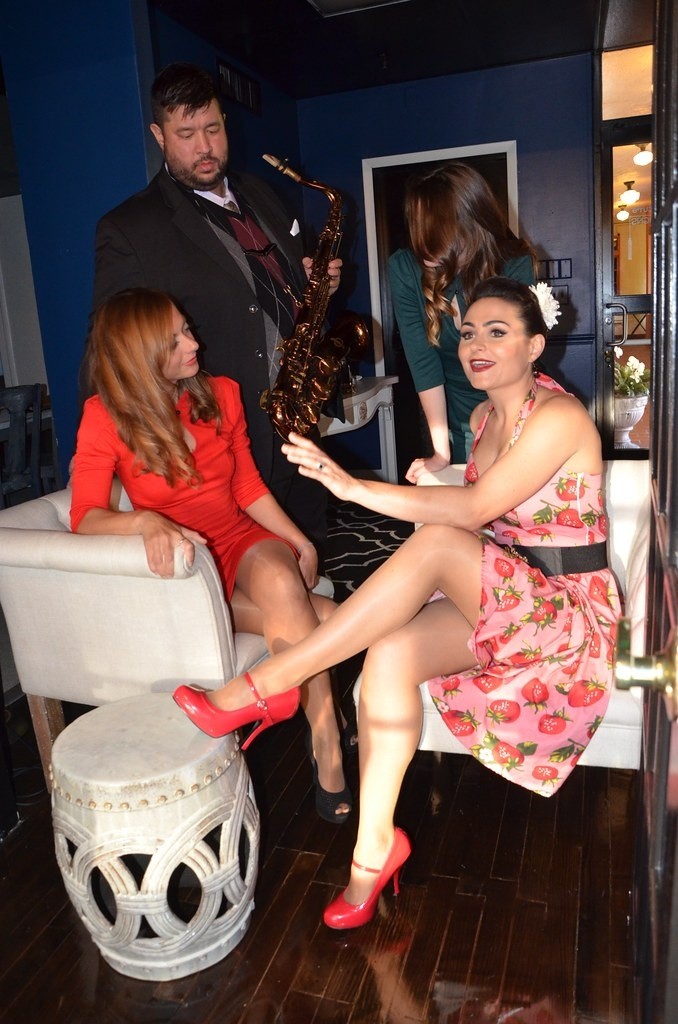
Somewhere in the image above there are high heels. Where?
[341,721,358,757]
[172,671,300,751]
[313,753,353,823]
[323,827,411,930]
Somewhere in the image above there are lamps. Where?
[616,205,630,221]
[620,181,641,204]
[634,143,654,166]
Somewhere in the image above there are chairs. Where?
[0,382,44,510]
[353,460,650,771]
[0,490,335,790]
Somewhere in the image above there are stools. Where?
[49,693,260,982]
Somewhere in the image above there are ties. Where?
[227,201,241,215]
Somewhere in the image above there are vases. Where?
[615,397,648,450]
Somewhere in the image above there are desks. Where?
[315,375,402,484]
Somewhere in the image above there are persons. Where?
[171,280,625,930]
[70,66,540,826]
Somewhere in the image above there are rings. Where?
[319,463,326,470]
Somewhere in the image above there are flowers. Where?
[528,281,563,331]
[614,346,651,398]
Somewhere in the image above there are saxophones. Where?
[258,155,367,443]
